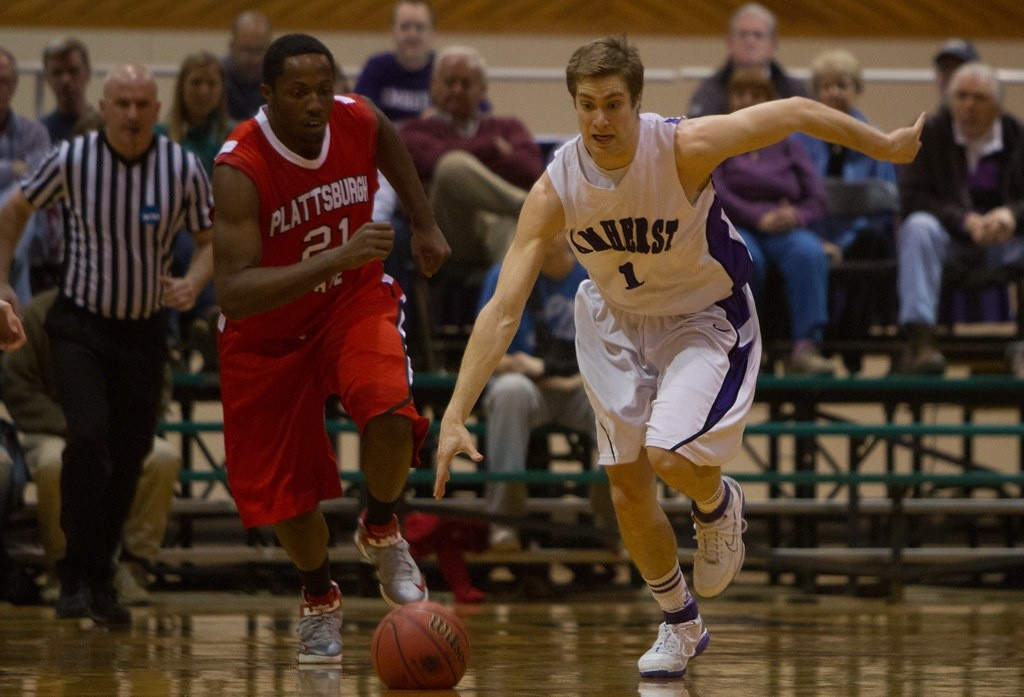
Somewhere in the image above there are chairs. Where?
[407,139,1022,378]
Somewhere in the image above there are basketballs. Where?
[370,601,471,689]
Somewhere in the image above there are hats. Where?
[932,38,977,62]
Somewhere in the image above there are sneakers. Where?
[297,663,341,697]
[55,579,132,630]
[139,612,173,635]
[692,476,746,600]
[111,564,154,606]
[637,615,709,677]
[637,679,689,697]
[351,508,430,609]
[486,519,522,552]
[296,580,343,663]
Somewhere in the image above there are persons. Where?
[0,47,51,303]
[212,33,452,663]
[477,229,615,555]
[788,49,896,322]
[433,37,928,677]
[895,39,1024,378]
[0,62,216,628]
[31,35,100,296]
[155,50,239,372]
[710,69,837,378]
[684,5,802,120]
[221,8,273,121]
[0,284,182,605]
[354,0,491,123]
[395,45,546,262]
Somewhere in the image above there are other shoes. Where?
[791,345,834,375]
[894,341,943,376]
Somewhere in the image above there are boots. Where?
[437,539,482,602]
